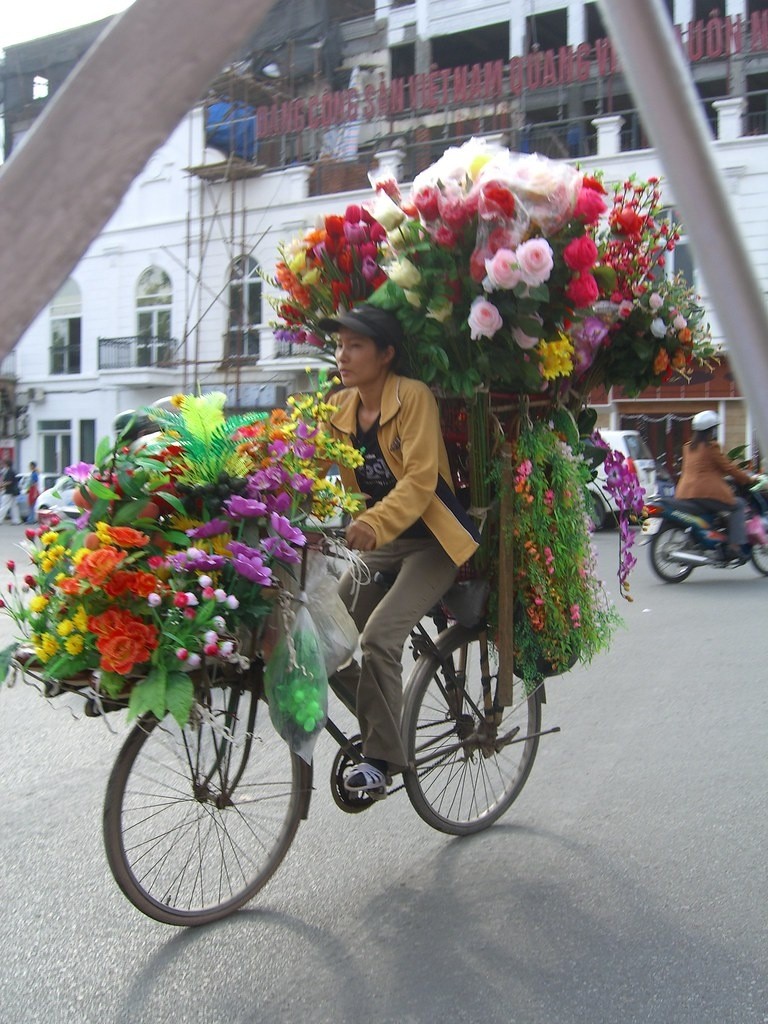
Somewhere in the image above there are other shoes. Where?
[728,546,750,559]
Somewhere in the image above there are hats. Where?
[317,305,402,349]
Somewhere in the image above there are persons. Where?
[308,306,483,792]
[673,410,760,560]
[1,460,22,525]
[23,462,39,524]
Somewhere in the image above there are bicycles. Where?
[87,519,563,922]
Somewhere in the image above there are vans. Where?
[578,428,663,533]
[0,471,63,515]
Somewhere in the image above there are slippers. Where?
[343,763,392,790]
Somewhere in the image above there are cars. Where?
[33,471,91,524]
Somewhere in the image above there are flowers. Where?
[1,130,724,731]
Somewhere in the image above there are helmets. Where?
[690,411,720,430]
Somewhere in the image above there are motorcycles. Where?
[634,466,768,588]
